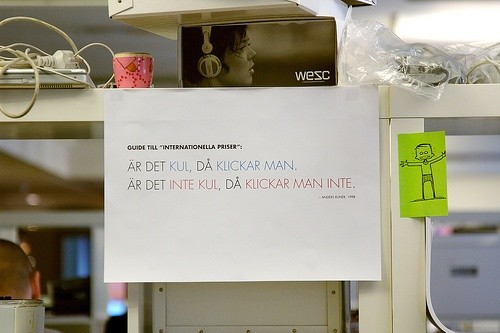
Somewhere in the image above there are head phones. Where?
[198,25,222,77]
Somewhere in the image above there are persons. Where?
[180,23,257,87]
[1,239,64,332]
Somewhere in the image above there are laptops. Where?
[1,68,96,89]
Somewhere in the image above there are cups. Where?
[112,50,154,88]
